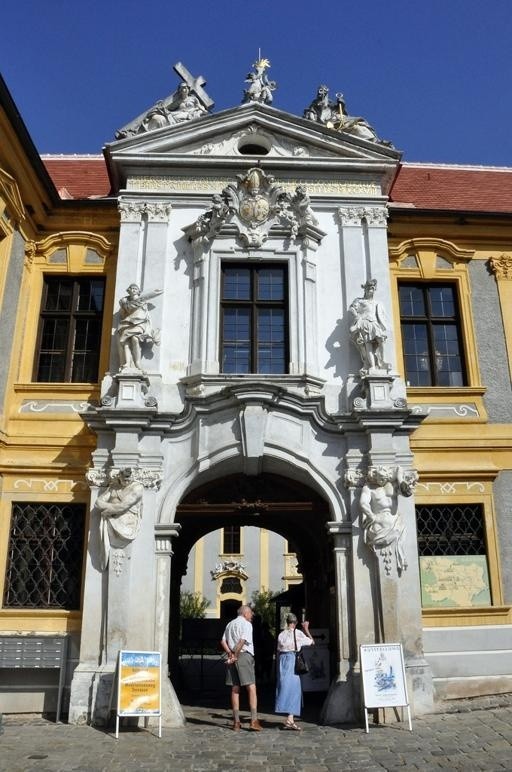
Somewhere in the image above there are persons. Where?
[348,280,391,372]
[308,83,377,141]
[273,611,319,728]
[360,464,414,545]
[118,287,160,373]
[97,466,143,567]
[245,70,262,101]
[189,184,320,252]
[221,603,263,730]
[146,83,207,132]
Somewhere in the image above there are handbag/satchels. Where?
[294,628,309,675]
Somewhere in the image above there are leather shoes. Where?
[234,720,242,731]
[249,719,264,731]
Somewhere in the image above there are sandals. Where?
[286,719,301,730]
